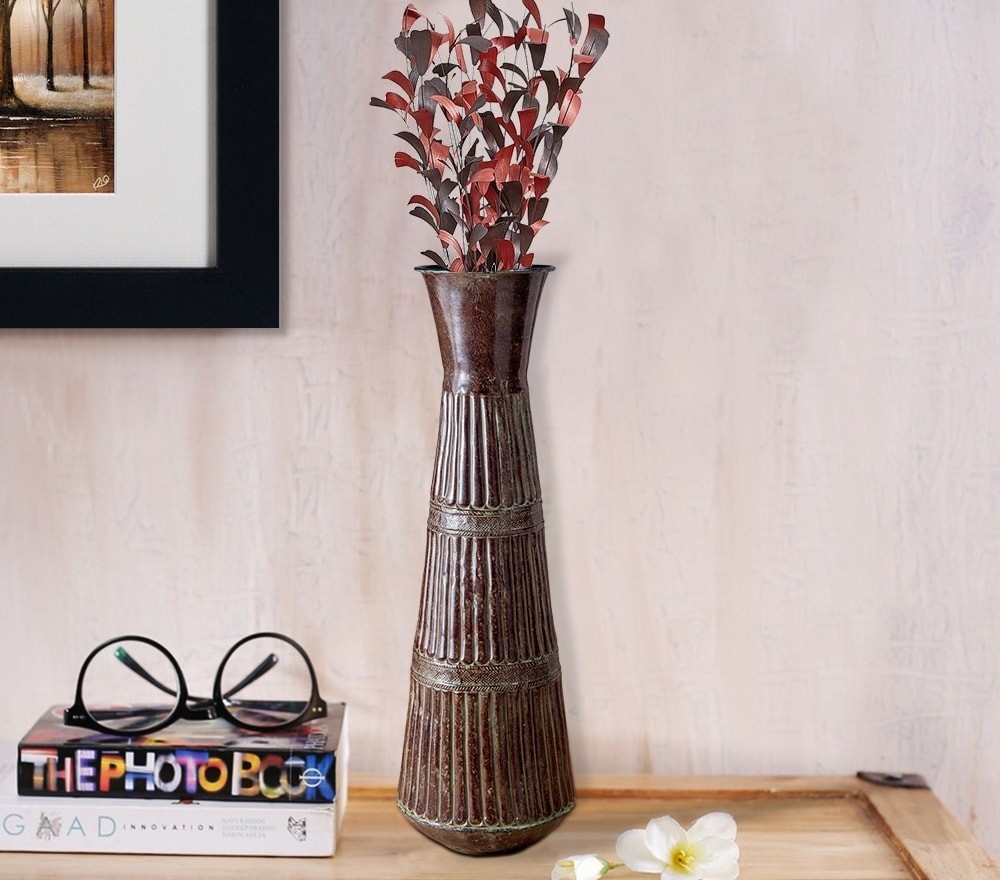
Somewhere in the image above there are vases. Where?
[398,257,575,855]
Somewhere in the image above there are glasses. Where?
[63,631,328,738]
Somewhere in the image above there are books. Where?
[17,702,351,803]
[0,764,349,859]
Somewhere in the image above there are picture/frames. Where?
[0,0,284,333]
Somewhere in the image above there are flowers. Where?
[612,812,757,880]
[549,851,626,880]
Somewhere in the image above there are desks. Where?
[0,769,1000,880]
